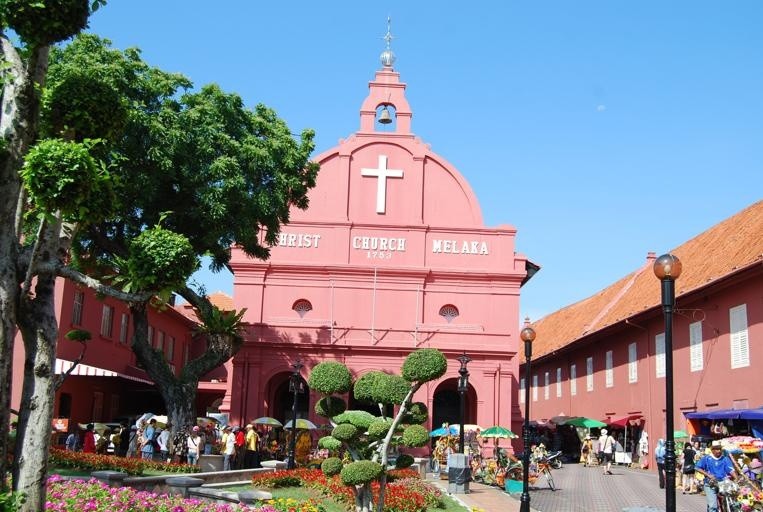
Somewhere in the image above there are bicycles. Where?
[709,481,739,512]
[503,455,555,491]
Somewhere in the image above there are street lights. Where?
[456,353,471,455]
[519,328,535,512]
[653,253,682,511]
[287,361,304,469]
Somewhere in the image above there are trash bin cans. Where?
[447,454,470,494]
[200,454,224,472]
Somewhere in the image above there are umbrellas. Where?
[430,422,524,459]
[136,410,168,427]
[284,418,318,429]
[565,417,607,438]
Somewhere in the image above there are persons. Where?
[599,429,616,474]
[581,437,593,466]
[655,439,667,488]
[681,443,696,494]
[64,418,311,466]
[692,441,701,459]
[694,441,738,512]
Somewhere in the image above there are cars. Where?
[88,422,124,454]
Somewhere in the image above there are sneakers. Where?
[682,491,693,495]
[603,470,613,475]
[583,462,589,468]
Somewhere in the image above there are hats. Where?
[86,424,94,431]
[600,428,608,436]
[246,424,254,428]
[131,425,140,430]
[221,424,230,431]
[685,443,692,449]
[584,436,591,440]
[232,426,240,433]
[155,428,161,433]
[166,422,174,427]
[710,440,725,449]
[192,426,200,431]
